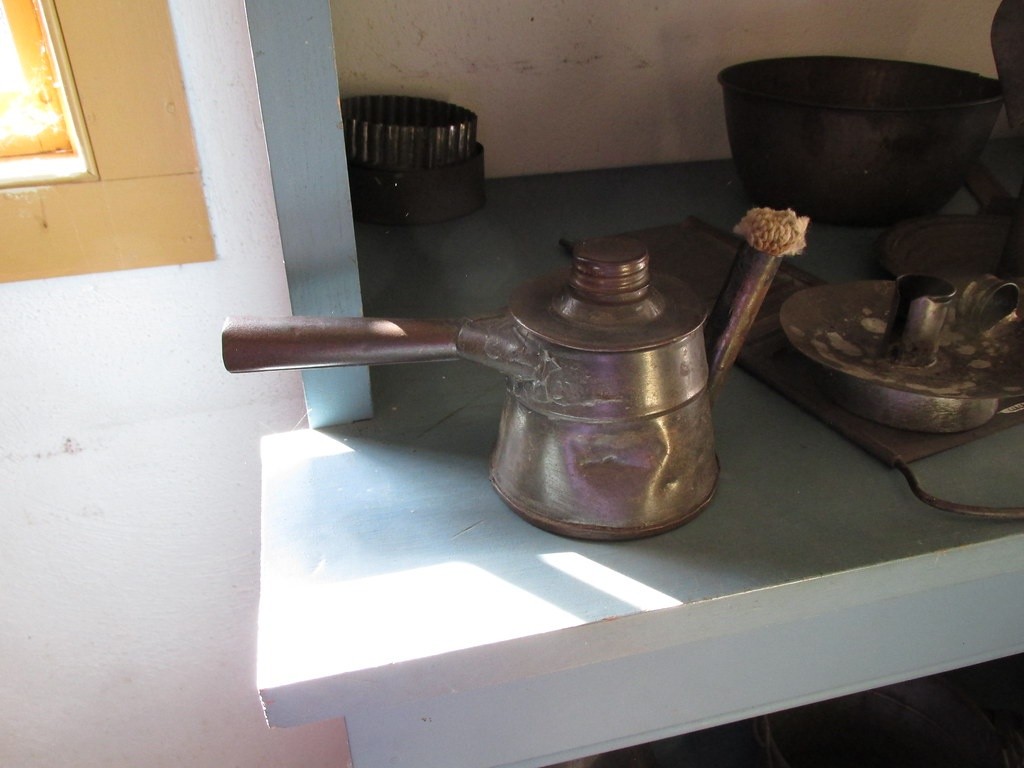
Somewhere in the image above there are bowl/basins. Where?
[717,54,1005,229]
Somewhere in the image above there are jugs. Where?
[221,208,808,541]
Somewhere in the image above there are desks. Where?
[253,157,1024,768]
